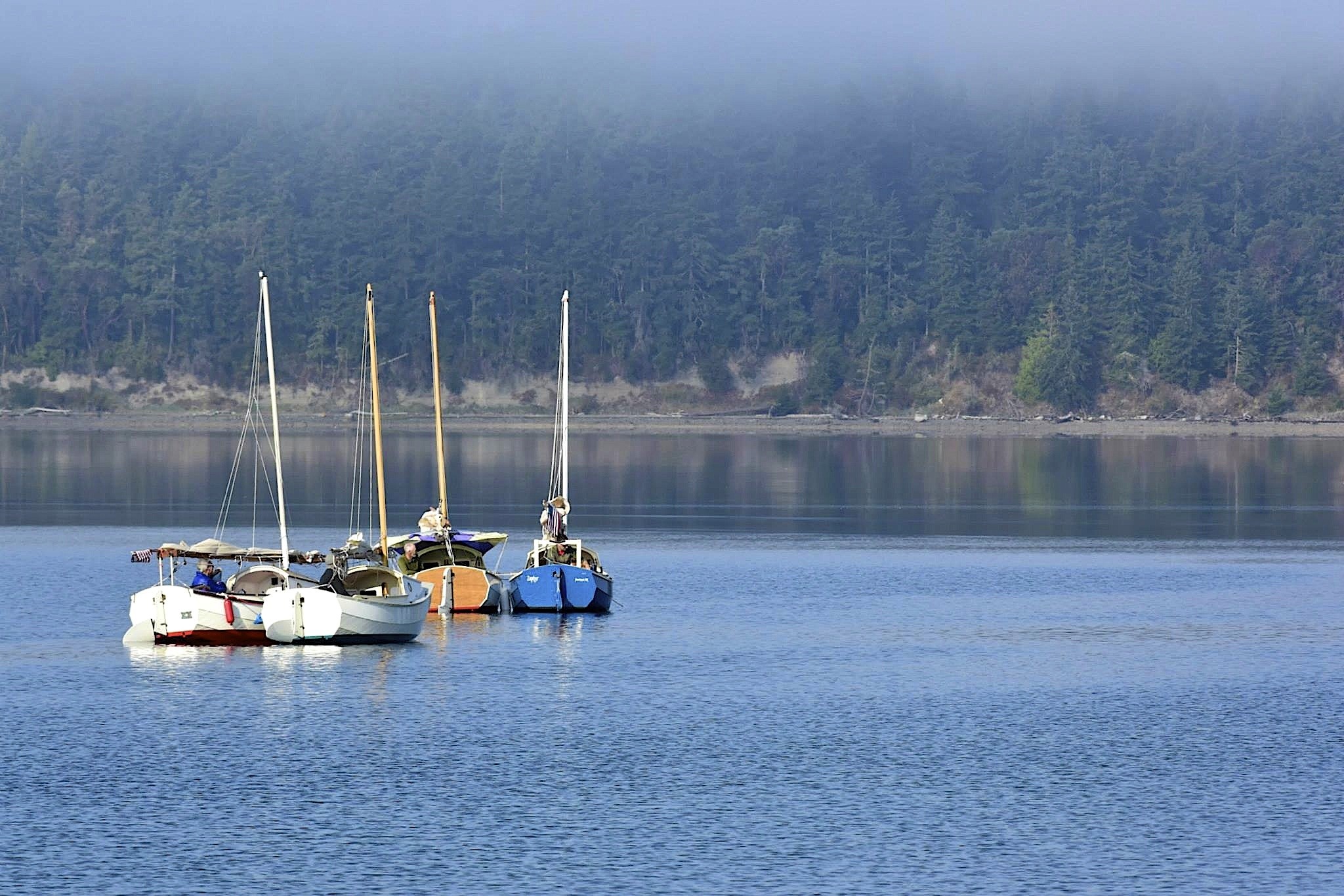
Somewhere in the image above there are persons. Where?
[547,544,576,566]
[398,543,419,575]
[191,559,226,594]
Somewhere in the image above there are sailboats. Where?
[508,288,614,615]
[383,290,507,613]
[129,269,437,646]
